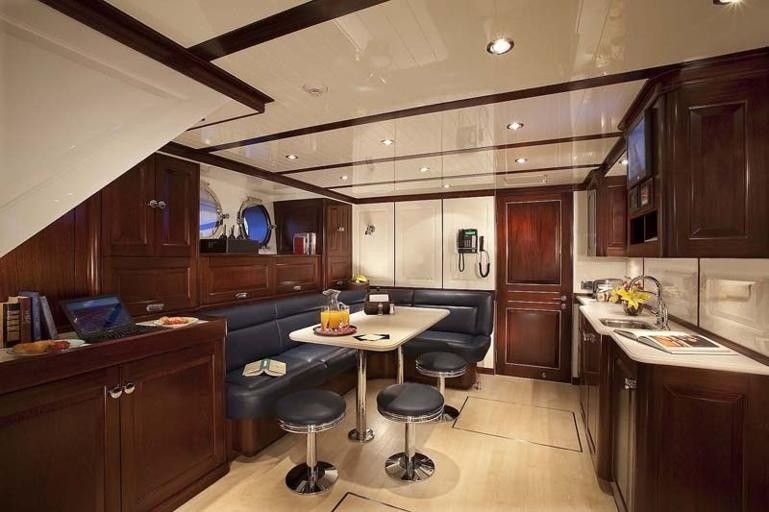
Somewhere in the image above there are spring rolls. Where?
[159,315,189,325]
[11,339,70,355]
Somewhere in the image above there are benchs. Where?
[199,286,493,458]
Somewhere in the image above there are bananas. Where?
[350,273,368,284]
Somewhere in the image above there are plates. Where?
[7,338,86,357]
[154,317,199,328]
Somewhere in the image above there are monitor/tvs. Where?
[625,108,654,190]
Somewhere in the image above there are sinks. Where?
[597,316,658,332]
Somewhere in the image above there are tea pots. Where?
[322,289,346,311]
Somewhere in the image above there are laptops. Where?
[58,294,176,343]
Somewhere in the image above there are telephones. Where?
[458,229,477,253]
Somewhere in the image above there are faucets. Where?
[627,274,669,332]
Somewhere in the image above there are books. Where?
[241,357,287,377]
[0,290,60,349]
[292,232,317,254]
[614,328,738,355]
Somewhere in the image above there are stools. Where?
[272,389,346,496]
[376,383,444,483]
[416,351,466,423]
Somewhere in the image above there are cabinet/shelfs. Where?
[273,257,322,295]
[100,151,199,257]
[0,318,228,512]
[198,256,274,305]
[577,302,769,512]
[584,54,769,258]
[101,256,199,317]
[273,198,350,256]
[327,257,351,286]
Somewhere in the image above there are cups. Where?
[319,307,349,332]
